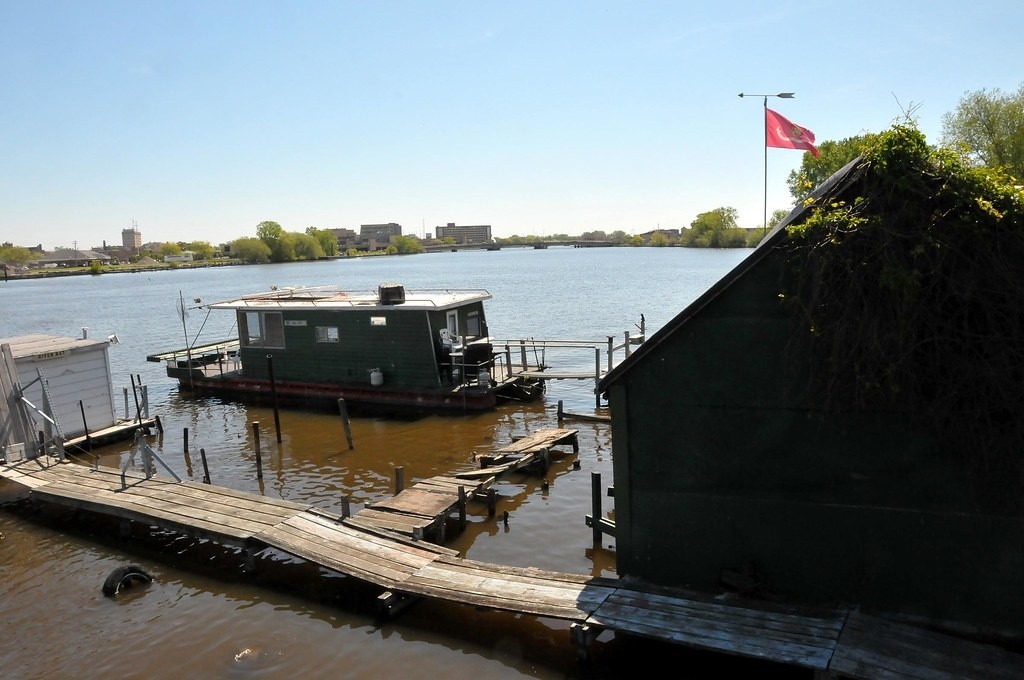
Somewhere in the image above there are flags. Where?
[766,107,820,159]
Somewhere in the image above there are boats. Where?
[145,282,552,412]
